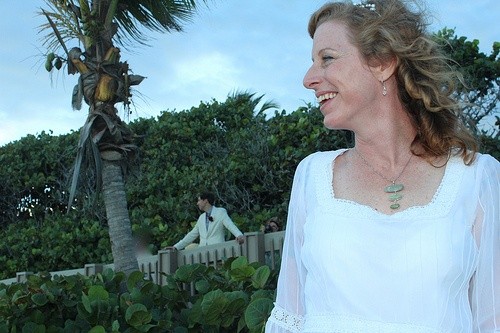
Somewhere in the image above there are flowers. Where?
[208,217,213,222]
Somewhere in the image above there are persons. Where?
[262,0,500,333]
[163,191,246,251]
[266,217,282,232]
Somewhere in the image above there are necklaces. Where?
[354,146,416,208]
